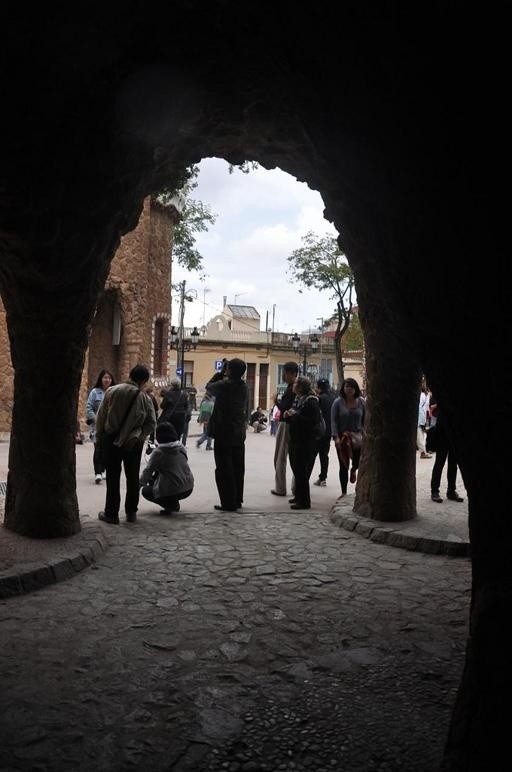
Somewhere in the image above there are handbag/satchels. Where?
[312,418,326,440]
[341,431,364,456]
[157,410,172,424]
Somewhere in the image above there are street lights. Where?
[202,288,243,327]
[316,315,325,382]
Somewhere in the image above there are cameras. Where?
[222,357,229,370]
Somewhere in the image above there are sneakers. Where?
[350,468,357,483]
[197,440,214,450]
[94,472,106,483]
[449,495,463,502]
[431,495,443,503]
[314,479,326,487]
[214,503,242,511]
[98,507,180,525]
[271,490,310,509]
[420,452,432,458]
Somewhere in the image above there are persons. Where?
[250,407,268,434]
[431,405,464,503]
[330,378,366,500]
[85,369,116,484]
[314,378,336,487]
[205,358,250,510]
[139,421,194,513]
[143,377,217,456]
[269,361,302,497]
[416,380,439,459]
[283,375,326,511]
[93,364,157,524]
[270,399,282,436]
